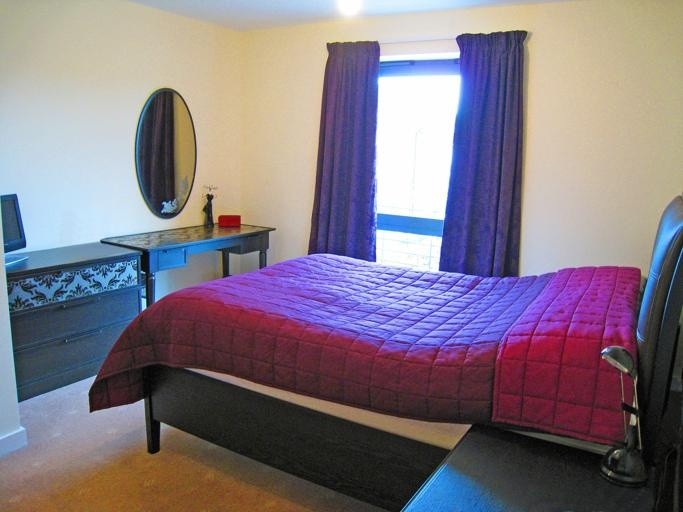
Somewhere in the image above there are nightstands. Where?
[398,423,683,511]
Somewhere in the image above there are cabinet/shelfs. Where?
[3,241,143,402]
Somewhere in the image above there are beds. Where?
[143,195,683,512]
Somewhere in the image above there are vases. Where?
[203,195,214,228]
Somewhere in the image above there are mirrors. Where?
[135,88,198,219]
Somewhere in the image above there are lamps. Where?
[599,341,652,489]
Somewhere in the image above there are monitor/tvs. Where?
[0,194,29,267]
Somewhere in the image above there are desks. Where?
[100,223,277,308]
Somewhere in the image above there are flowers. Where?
[161,199,178,214]
[203,184,218,196]
